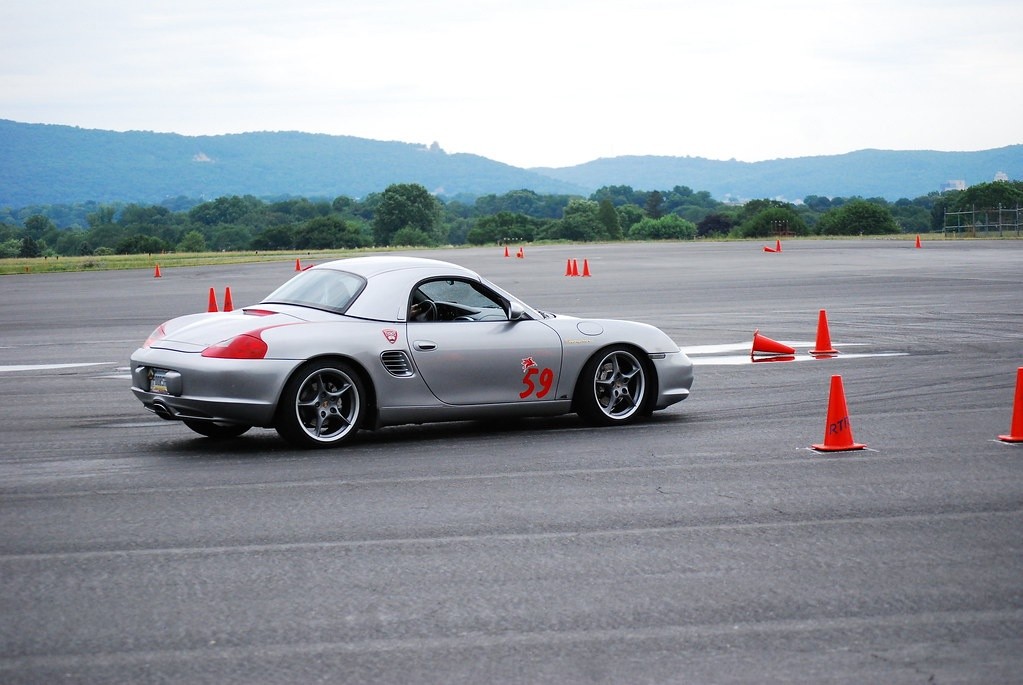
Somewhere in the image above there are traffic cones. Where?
[810,376,868,453]
[810,308,838,353]
[565,259,572,276]
[582,259,591,276]
[751,329,795,359]
[571,259,579,276]
[516,246,523,258]
[913,234,921,248]
[997,367,1023,442]
[154,264,161,278]
[763,246,776,252]
[775,240,783,252]
[208,288,218,312]
[223,286,234,312]
[504,247,509,256]
[296,259,300,271]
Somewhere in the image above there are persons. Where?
[409,304,421,319]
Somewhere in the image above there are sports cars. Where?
[129,256,693,450]
